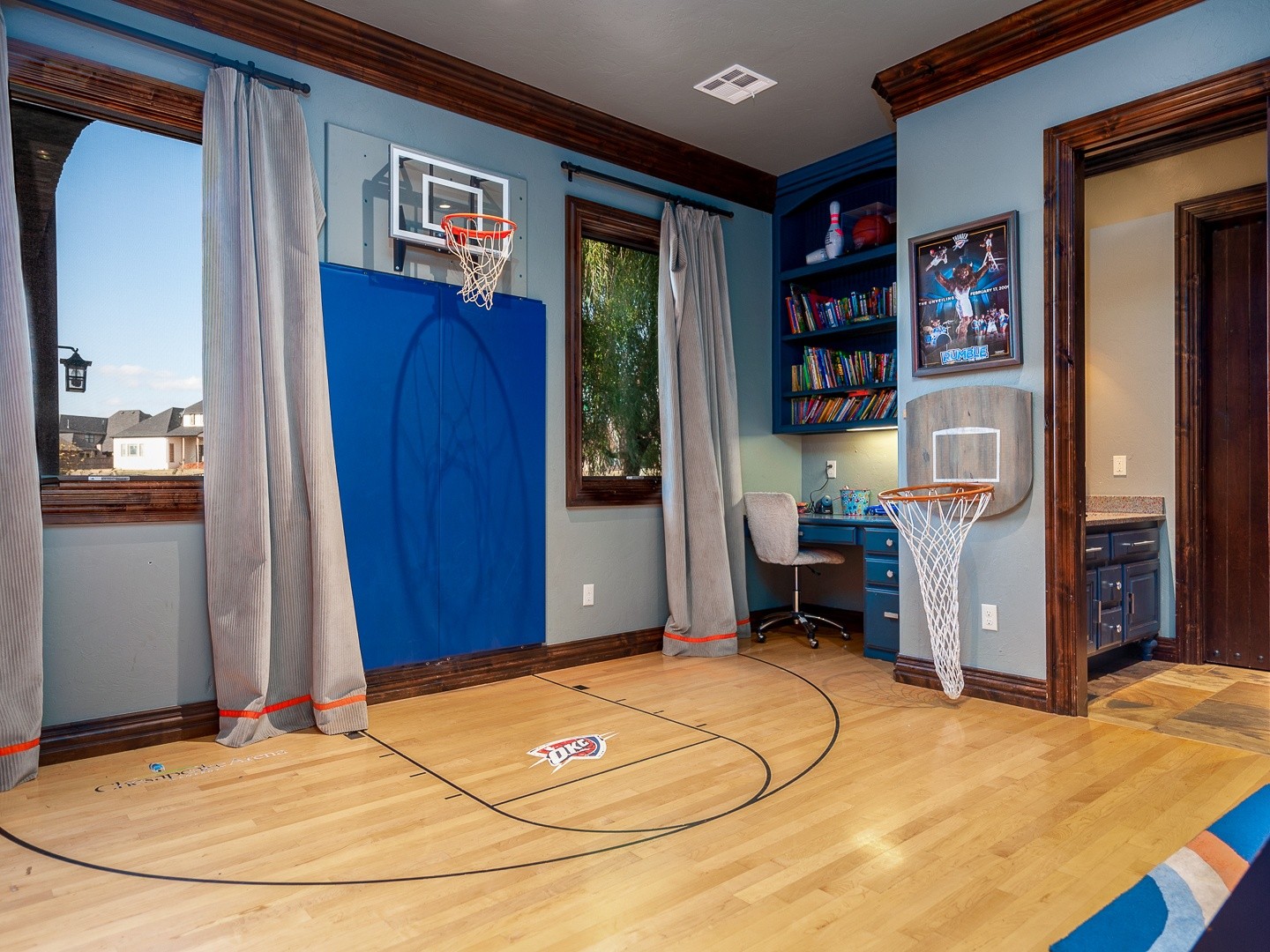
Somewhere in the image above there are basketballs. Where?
[852,214,890,248]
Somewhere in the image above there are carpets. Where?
[1048,778,1270,952]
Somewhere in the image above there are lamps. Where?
[57,344,92,393]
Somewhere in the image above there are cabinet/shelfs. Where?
[770,131,897,437]
[1086,521,1161,664]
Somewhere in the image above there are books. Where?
[784,283,897,334]
[791,388,897,426]
[791,346,897,391]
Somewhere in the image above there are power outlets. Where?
[1112,455,1127,476]
[583,584,594,606]
[981,603,998,631]
[826,460,837,478]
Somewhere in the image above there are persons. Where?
[921,307,1009,347]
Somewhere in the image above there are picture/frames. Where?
[906,209,1023,378]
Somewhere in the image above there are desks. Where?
[742,511,899,664]
